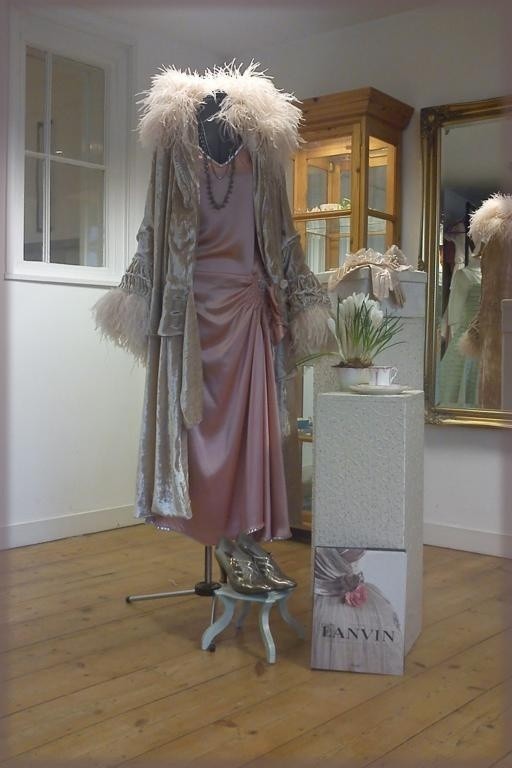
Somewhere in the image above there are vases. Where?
[332,366,373,390]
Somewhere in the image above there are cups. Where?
[369,365,398,386]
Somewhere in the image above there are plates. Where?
[349,383,408,395]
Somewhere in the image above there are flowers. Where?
[285,290,407,379]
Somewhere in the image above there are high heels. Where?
[212,534,297,594]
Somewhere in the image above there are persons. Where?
[449,258,465,289]
[95,60,335,550]
[458,194,512,410]
[314,546,406,676]
[434,253,486,410]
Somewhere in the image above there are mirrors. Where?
[418,94,512,431]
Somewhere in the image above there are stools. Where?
[200,583,307,664]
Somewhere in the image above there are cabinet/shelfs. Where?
[284,87,414,533]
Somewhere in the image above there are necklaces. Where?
[199,123,238,210]
